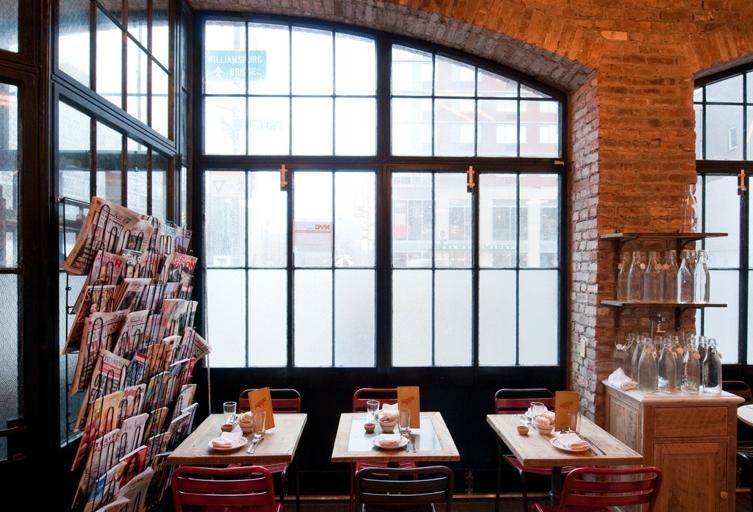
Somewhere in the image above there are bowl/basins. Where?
[534,422,554,435]
[379,416,397,432]
[238,420,253,434]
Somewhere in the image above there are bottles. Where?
[678,183,698,233]
[612,330,723,394]
[616,249,710,303]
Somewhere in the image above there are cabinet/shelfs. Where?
[600,380,746,511]
[597,231,729,330]
[59,196,197,512]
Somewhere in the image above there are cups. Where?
[221,425,232,432]
[531,402,545,424]
[517,426,529,436]
[222,401,236,427]
[364,424,375,433]
[399,411,411,437]
[254,410,267,434]
[567,410,581,433]
[367,400,379,425]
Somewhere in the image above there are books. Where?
[62,195,212,512]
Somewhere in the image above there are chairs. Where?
[170,463,283,512]
[350,387,417,507]
[356,465,453,512]
[494,388,577,511]
[532,467,663,512]
[225,387,301,512]
[722,381,752,506]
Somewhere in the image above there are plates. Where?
[372,434,407,450]
[208,436,248,451]
[550,437,590,452]
[525,410,556,418]
